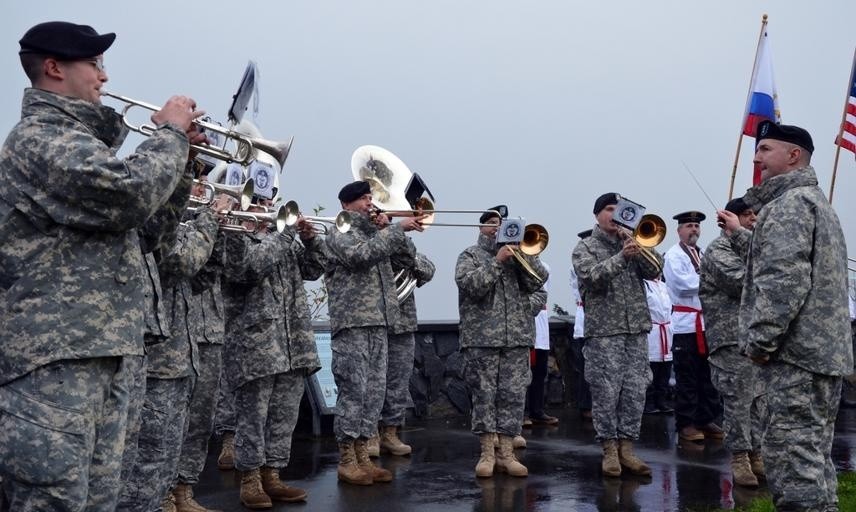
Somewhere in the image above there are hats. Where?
[725,197,751,215]
[756,120,815,153]
[592,192,622,215]
[338,180,371,203]
[18,21,116,59]
[195,158,217,177]
[250,185,279,204]
[673,211,707,224]
[479,205,509,224]
[577,228,594,241]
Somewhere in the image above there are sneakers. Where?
[701,423,726,440]
[680,426,705,442]
[522,415,533,426]
[643,402,659,416]
[659,402,677,413]
[581,410,592,419]
[535,414,559,425]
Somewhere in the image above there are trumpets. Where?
[300,211,351,236]
[182,207,287,235]
[98,87,295,174]
[189,177,256,211]
[249,201,298,227]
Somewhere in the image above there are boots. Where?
[261,468,308,502]
[217,432,235,470]
[337,439,372,486]
[620,437,652,476]
[239,468,272,508]
[751,446,765,475]
[156,493,176,511]
[475,433,496,478]
[732,451,760,489]
[600,439,621,476]
[496,433,529,477]
[367,433,381,456]
[176,481,215,511]
[511,434,526,449]
[380,426,412,456]
[355,434,393,483]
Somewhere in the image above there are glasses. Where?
[72,56,106,71]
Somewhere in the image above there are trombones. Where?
[614,215,666,276]
[495,225,548,283]
[366,198,502,228]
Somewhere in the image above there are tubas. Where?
[349,145,419,307]
